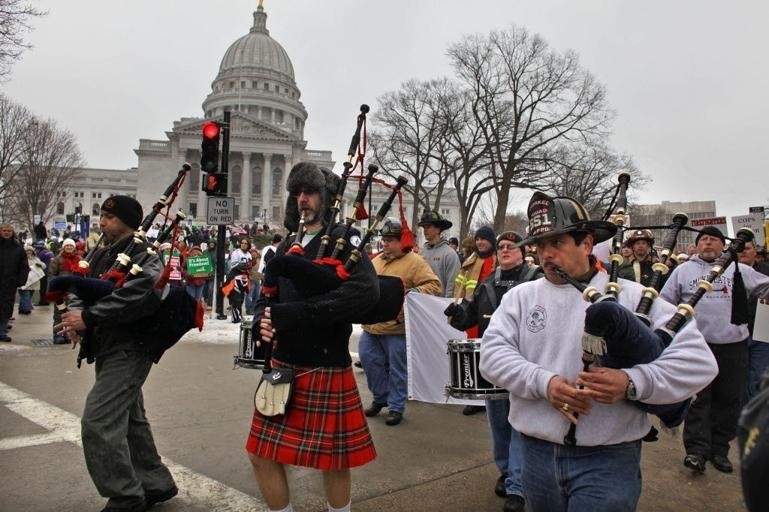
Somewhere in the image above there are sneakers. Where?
[711,454,733,473]
[463,406,486,415]
[504,495,524,512]
[145,487,179,507]
[385,411,401,425]
[364,401,386,416]
[100,500,147,512]
[54,336,71,344]
[685,454,705,471]
[496,474,508,497]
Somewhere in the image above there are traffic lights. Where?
[201,122,220,169]
[204,175,226,195]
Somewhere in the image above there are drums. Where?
[235,314,268,370]
[443,337,513,400]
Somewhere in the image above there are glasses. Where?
[292,188,316,198]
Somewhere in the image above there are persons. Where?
[734,388,769,511]
[52,194,177,511]
[478,189,720,511]
[244,160,380,511]
[660,225,769,475]
[0,161,769,426]
[443,232,543,512]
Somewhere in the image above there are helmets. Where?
[418,211,452,229]
[510,192,617,247]
[378,220,403,237]
[629,229,654,243]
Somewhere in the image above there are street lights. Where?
[74,208,80,233]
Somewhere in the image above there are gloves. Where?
[444,302,465,320]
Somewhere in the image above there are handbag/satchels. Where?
[254,369,295,417]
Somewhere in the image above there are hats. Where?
[696,227,725,246]
[283,163,340,232]
[101,196,143,229]
[476,226,496,245]
[496,231,525,251]
[62,239,76,249]
